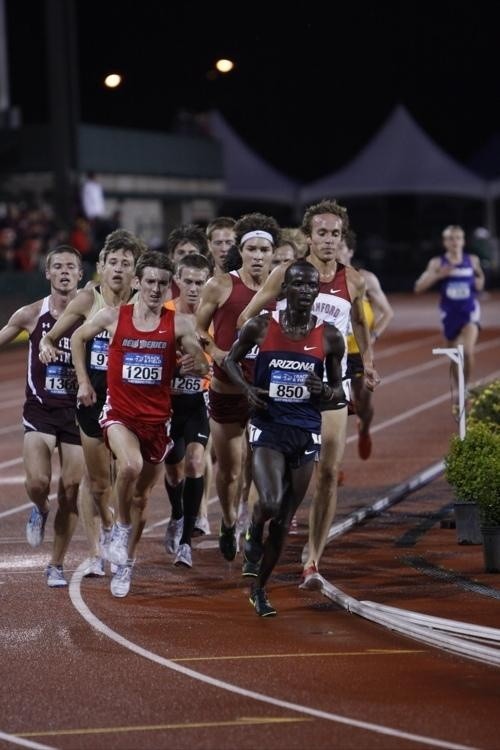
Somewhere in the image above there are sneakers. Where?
[172,543,193,570]
[26,496,49,547]
[288,514,297,536]
[243,522,263,564]
[83,557,105,577]
[241,560,259,579]
[109,564,131,598]
[98,507,115,558]
[297,561,324,591]
[236,510,250,536]
[194,517,211,536]
[47,563,67,588]
[249,587,277,617]
[357,418,372,461]
[219,516,237,561]
[108,522,131,567]
[165,513,184,554]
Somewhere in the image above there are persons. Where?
[1,167,123,269]
[414,222,486,420]
[0,197,393,617]
